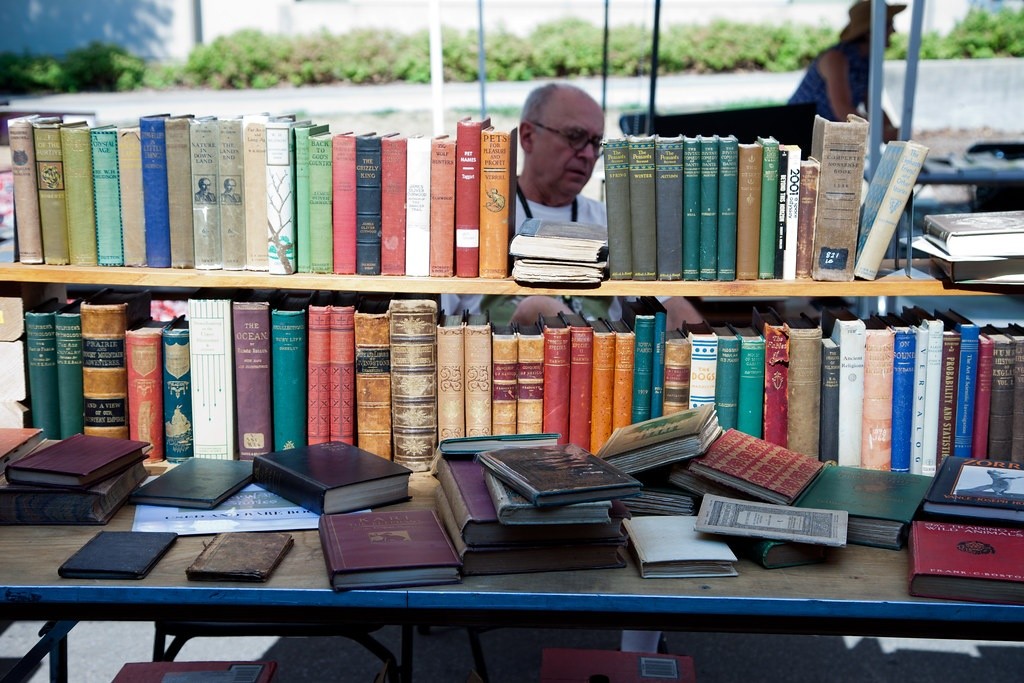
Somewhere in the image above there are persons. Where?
[442,83,702,654]
[789,0,897,143]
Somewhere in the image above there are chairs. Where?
[154,617,410,683]
[967,142,1024,213]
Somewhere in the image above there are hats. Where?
[839,1,908,41]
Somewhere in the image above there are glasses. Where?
[527,119,605,157]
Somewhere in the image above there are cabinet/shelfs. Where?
[0,263,1024,683]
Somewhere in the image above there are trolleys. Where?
[914,142,1024,212]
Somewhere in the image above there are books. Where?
[9,114,928,285]
[112,661,279,683]
[899,212,1024,285]
[541,648,696,683]
[0,296,1024,604]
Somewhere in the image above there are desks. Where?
[863,161,1024,258]
